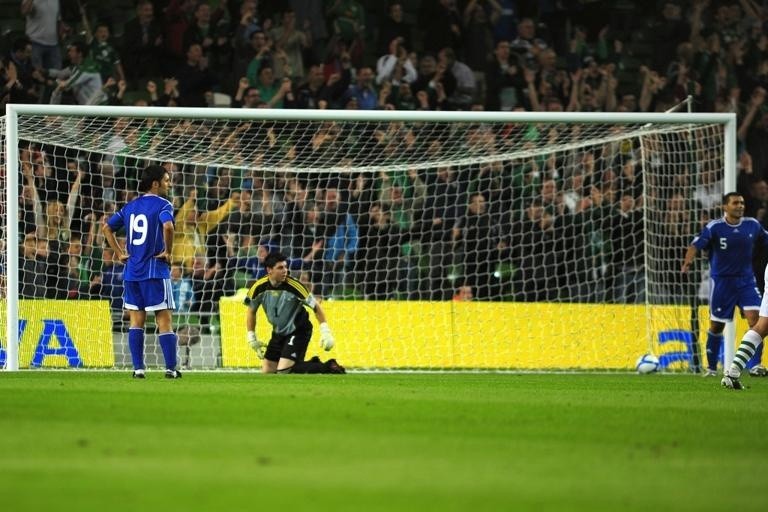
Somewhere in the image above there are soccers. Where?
[636,354,660,375]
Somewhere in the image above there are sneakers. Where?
[720,375,743,390]
[703,368,717,378]
[749,364,766,376]
[310,356,344,374]
[165,369,182,378]
[133,369,146,377]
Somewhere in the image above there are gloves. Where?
[248,331,268,360]
[319,322,334,351]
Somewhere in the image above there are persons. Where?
[679,192,768,376]
[0,1,768,335]
[102,164,184,378]
[721,264,768,390]
[244,252,348,375]
